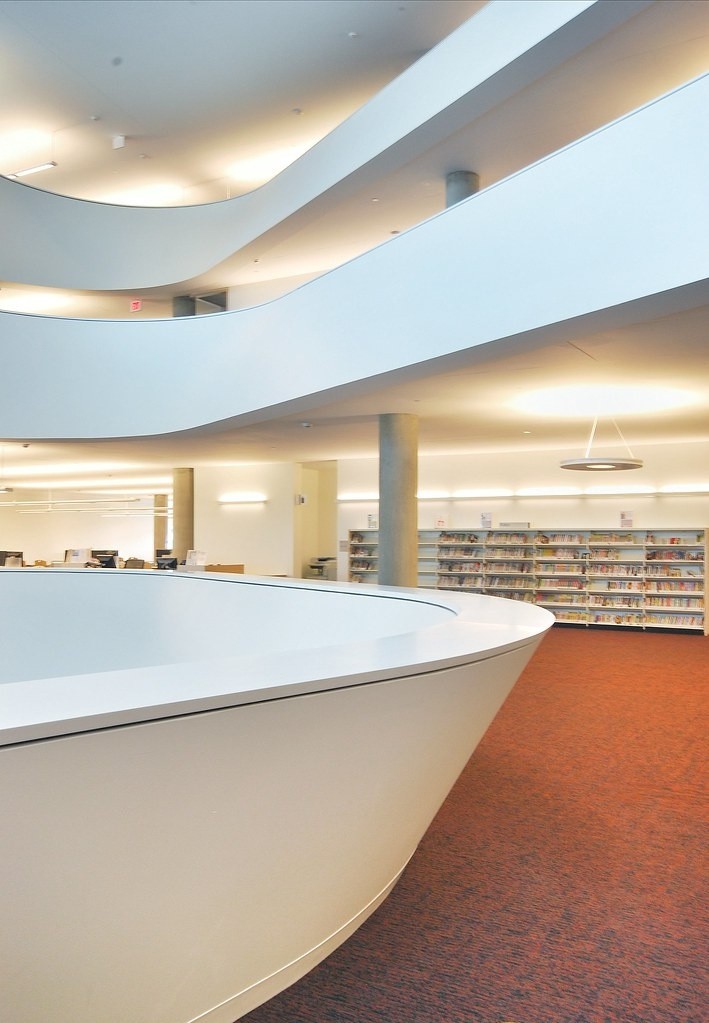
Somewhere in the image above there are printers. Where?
[307,556,337,581]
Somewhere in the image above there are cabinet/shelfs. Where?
[341,527,709,638]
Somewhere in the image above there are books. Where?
[438,533,704,629]
[351,533,373,583]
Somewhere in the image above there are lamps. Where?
[560,416,644,472]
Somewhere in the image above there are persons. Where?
[86,558,100,568]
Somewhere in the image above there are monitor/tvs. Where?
[6,552,23,567]
[97,555,116,568]
[155,557,178,570]
